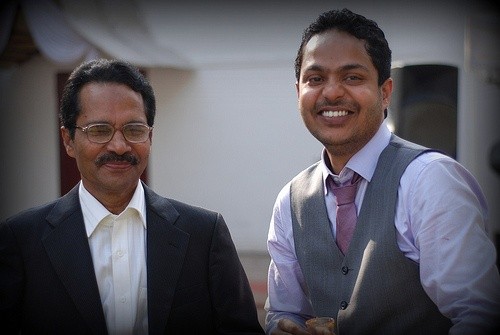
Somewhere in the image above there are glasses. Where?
[70,122,153,145]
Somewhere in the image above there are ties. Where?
[327,176,356,252]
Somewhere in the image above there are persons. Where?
[261,9,500,335]
[0,58,266,335]
